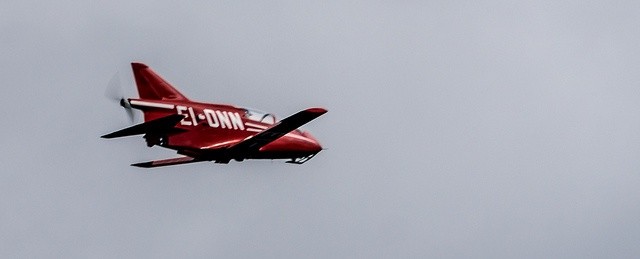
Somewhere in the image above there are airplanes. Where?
[101,62,329,169]
